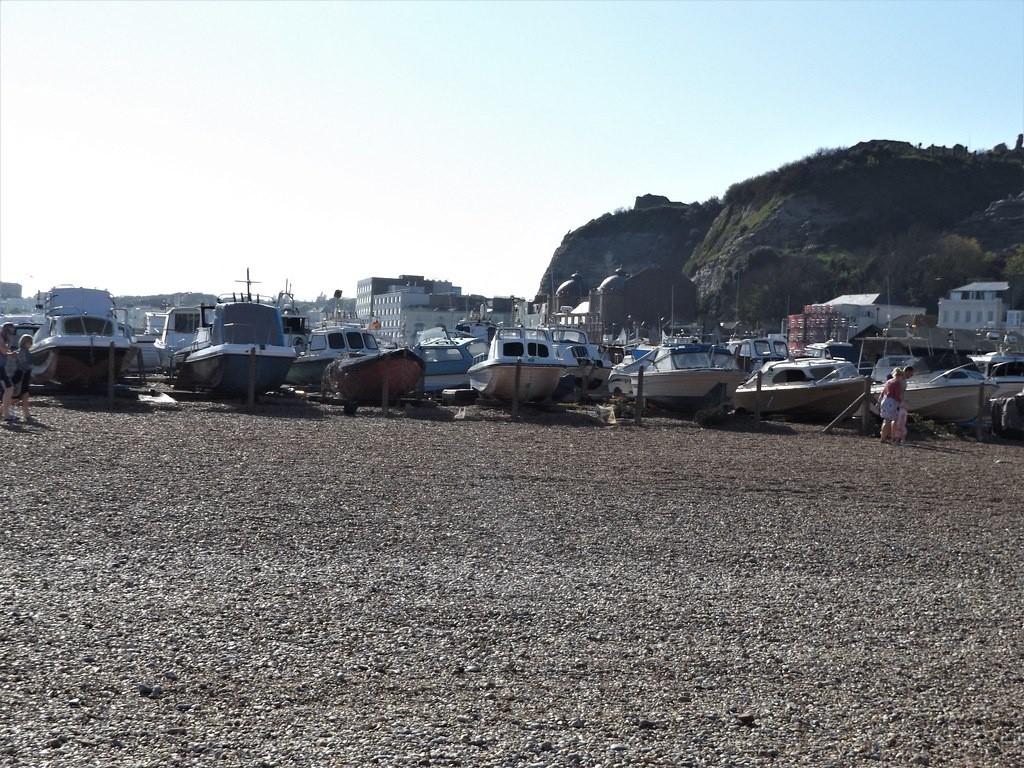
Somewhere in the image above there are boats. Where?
[0,268,1024,437]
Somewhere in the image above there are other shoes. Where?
[3,414,18,421]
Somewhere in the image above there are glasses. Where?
[28,342,32,345]
[10,332,15,335]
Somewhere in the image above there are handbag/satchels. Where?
[13,381,22,396]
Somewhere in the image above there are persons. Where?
[875,365,915,445]
[0,322,39,422]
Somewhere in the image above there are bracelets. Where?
[877,401,880,404]
